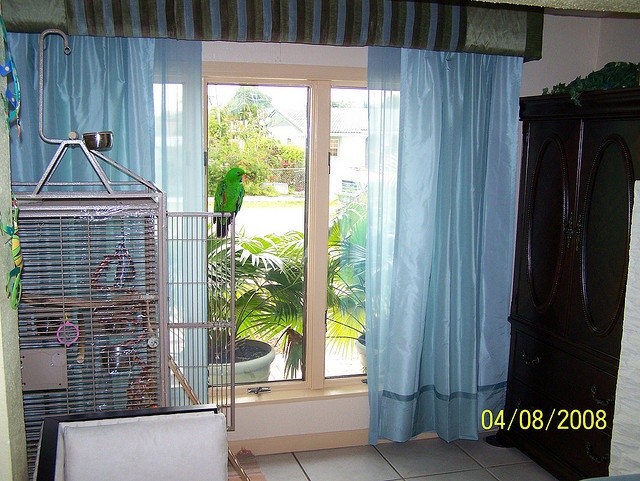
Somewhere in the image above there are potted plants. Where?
[327,201,368,376]
[208,234,305,384]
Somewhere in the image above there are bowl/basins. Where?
[84,131,112,149]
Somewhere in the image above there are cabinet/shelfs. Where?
[484,90,639,481]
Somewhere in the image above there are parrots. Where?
[212,167,247,239]
